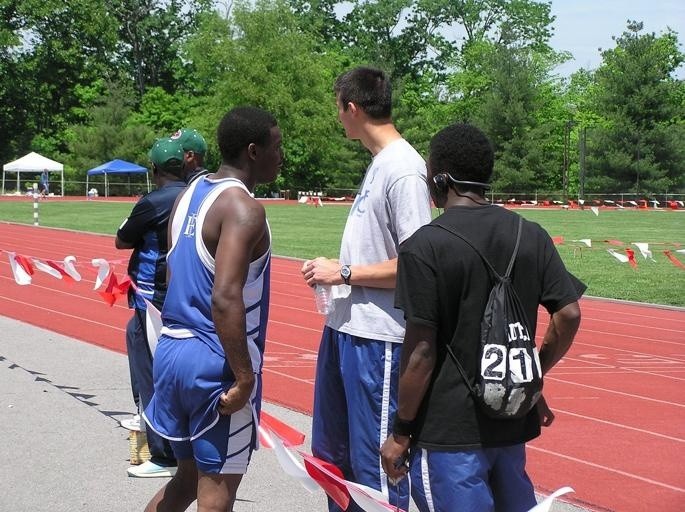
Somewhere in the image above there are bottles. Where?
[311,281,336,315]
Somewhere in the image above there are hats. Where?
[149,127,207,163]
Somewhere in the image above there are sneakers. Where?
[120,414,146,432]
[128,457,179,477]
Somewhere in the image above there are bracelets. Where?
[394,410,415,436]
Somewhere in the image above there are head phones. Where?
[432,171,492,194]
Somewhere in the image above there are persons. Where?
[143,109,284,512]
[300,67,434,512]
[40,169,49,195]
[119,129,207,433]
[114,137,191,479]
[380,123,588,512]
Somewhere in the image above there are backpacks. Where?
[471,281,545,416]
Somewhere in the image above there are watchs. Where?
[340,264,352,285]
